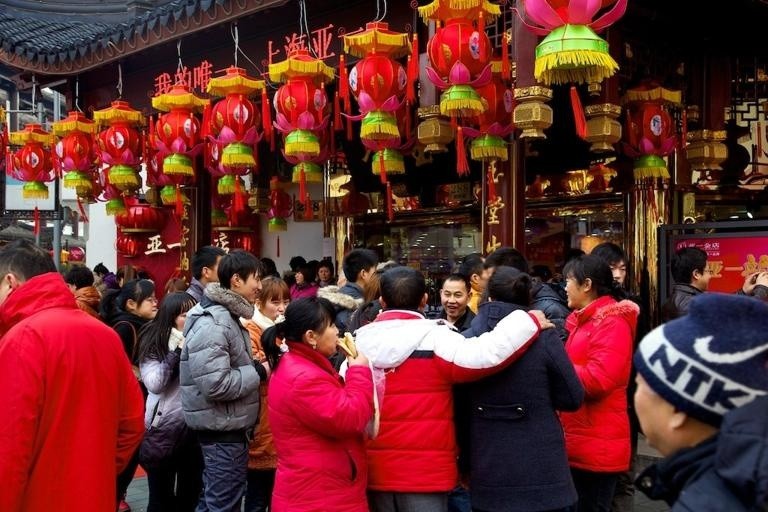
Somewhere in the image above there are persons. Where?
[0,238,145,510]
[65,244,639,511]
[634,291,767,510]
[652,247,766,324]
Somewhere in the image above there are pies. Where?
[337,332,357,359]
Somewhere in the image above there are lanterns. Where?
[2,1,680,258]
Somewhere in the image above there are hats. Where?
[633,293,768,428]
